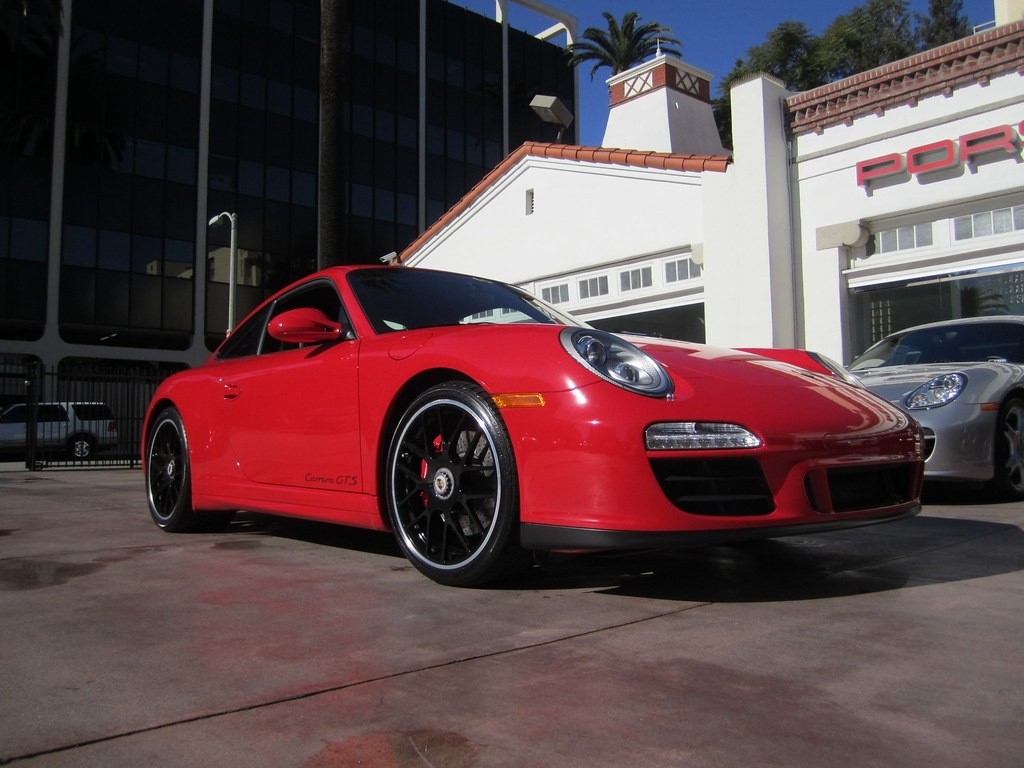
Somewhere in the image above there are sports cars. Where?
[142,265,927,588]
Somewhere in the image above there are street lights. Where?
[208,210,239,336]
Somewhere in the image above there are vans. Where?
[0,401,119,460]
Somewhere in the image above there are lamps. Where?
[530,94,575,142]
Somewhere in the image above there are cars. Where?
[840,315,1024,497]
